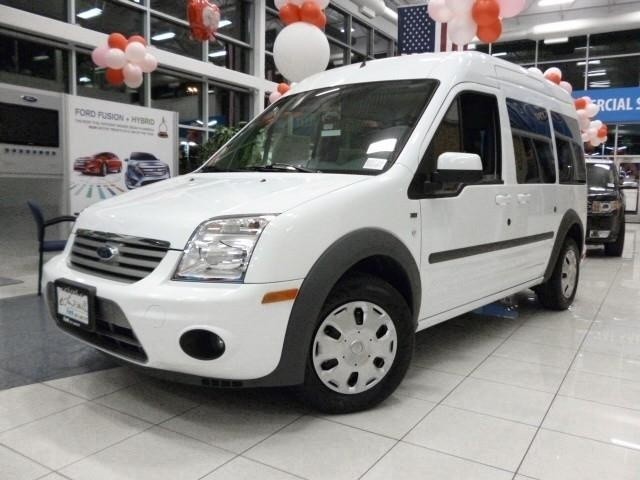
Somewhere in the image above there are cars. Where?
[72,152,122,175]
[124,151,171,189]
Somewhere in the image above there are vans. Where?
[41,49,592,414]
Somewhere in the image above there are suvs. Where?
[582,158,625,255]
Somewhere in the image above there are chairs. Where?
[26,198,79,296]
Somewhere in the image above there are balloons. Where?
[90,33,158,90]
[272,22,331,86]
[280,5,302,26]
[270,92,284,104]
[274,0,332,12]
[276,83,290,97]
[299,2,322,23]
[425,0,531,47]
[529,63,611,147]
[314,12,327,28]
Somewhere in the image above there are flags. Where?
[395,2,471,56]
